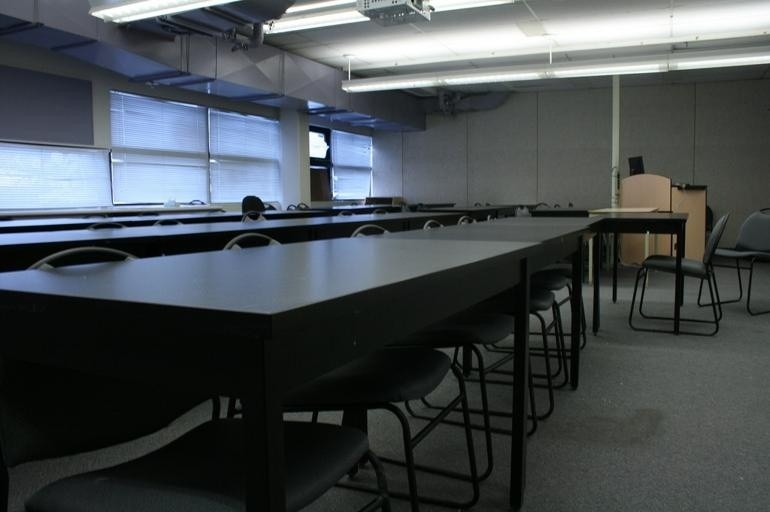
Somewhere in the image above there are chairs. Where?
[1,197,601,511]
[697,208,769,316]
[628,213,722,337]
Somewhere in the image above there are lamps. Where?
[90,2,235,24]
[341,37,770,94]
[262,0,511,35]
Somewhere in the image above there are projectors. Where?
[357,0,431,26]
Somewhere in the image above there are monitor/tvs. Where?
[629,157,644,175]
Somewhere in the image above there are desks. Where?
[593,212,688,336]
